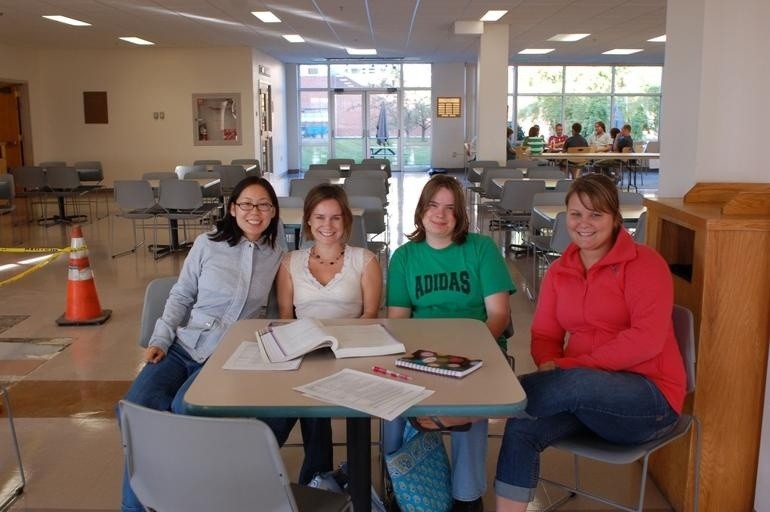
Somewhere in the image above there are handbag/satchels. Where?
[307,461,387,512]
[384,428,452,511]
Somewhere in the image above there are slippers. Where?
[408,417,471,432]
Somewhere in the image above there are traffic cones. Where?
[55,223,112,327]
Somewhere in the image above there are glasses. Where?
[234,202,273,211]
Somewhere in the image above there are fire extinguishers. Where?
[200,123,207,141]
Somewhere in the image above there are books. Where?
[255,316,406,365]
[395,348,484,378]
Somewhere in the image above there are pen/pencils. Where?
[371,365,415,381]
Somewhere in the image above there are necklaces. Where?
[310,247,344,266]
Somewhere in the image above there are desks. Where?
[183,319,530,512]
[369,146,420,165]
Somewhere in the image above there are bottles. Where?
[551,140,555,149]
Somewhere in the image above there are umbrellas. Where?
[375,100,390,159]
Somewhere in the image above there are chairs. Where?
[0,386,25,512]
[119,400,354,512]
[464,142,659,302]
[139,277,179,349]
[538,302,703,512]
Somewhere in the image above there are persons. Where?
[379,175,517,512]
[642,124,655,143]
[276,183,384,512]
[115,177,287,511]
[508,122,633,179]
[493,172,687,512]
